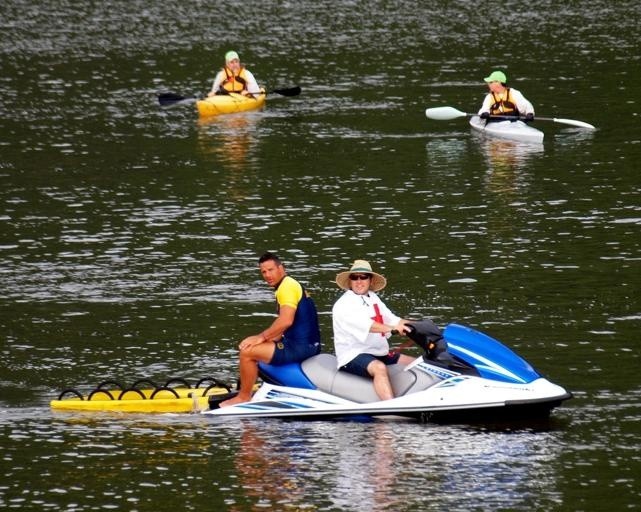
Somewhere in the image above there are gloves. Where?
[526,113,534,122]
[480,112,489,118]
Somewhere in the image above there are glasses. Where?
[349,274,368,280]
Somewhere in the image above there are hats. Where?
[225,50,239,62]
[336,260,387,292]
[484,71,507,84]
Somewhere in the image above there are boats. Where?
[196,88,266,117]
[469,116,544,144]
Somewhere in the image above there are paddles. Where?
[425,107,596,130]
[158,86,301,106]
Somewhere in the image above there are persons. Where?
[478,71,535,122]
[218,252,321,408]
[207,50,261,101]
[332,259,411,401]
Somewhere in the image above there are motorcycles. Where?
[202,318,572,424]
